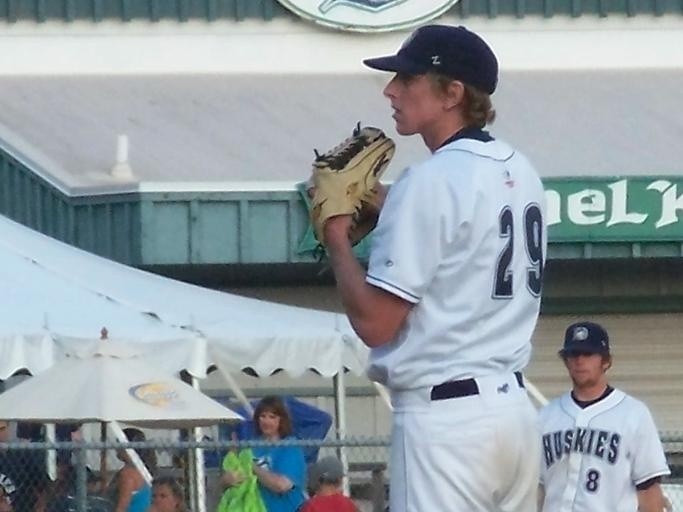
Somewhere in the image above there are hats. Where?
[315,456,347,481]
[560,322,609,354]
[364,24,496,93]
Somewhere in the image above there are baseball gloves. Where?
[298,127,396,253]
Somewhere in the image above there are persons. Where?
[0,418,191,512]
[217,395,309,512]
[295,456,359,512]
[535,321,671,512]
[305,23,548,512]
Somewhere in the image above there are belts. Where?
[430,372,523,400]
